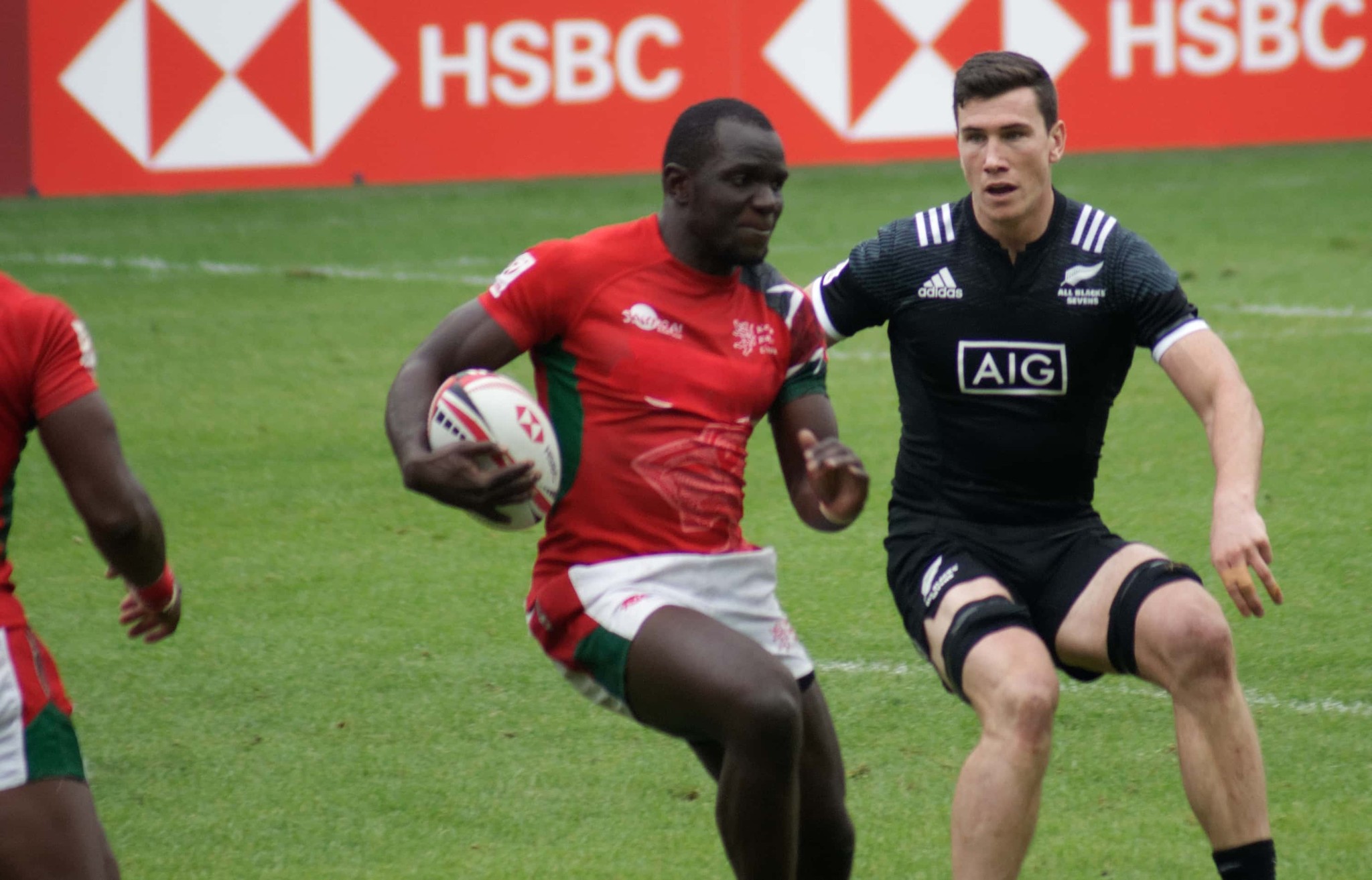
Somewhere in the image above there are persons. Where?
[790,53,1288,880]
[383,98,868,880]
[1,273,180,880]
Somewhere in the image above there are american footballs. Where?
[424,370,567,535]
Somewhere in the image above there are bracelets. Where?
[129,562,174,610]
[818,501,855,526]
